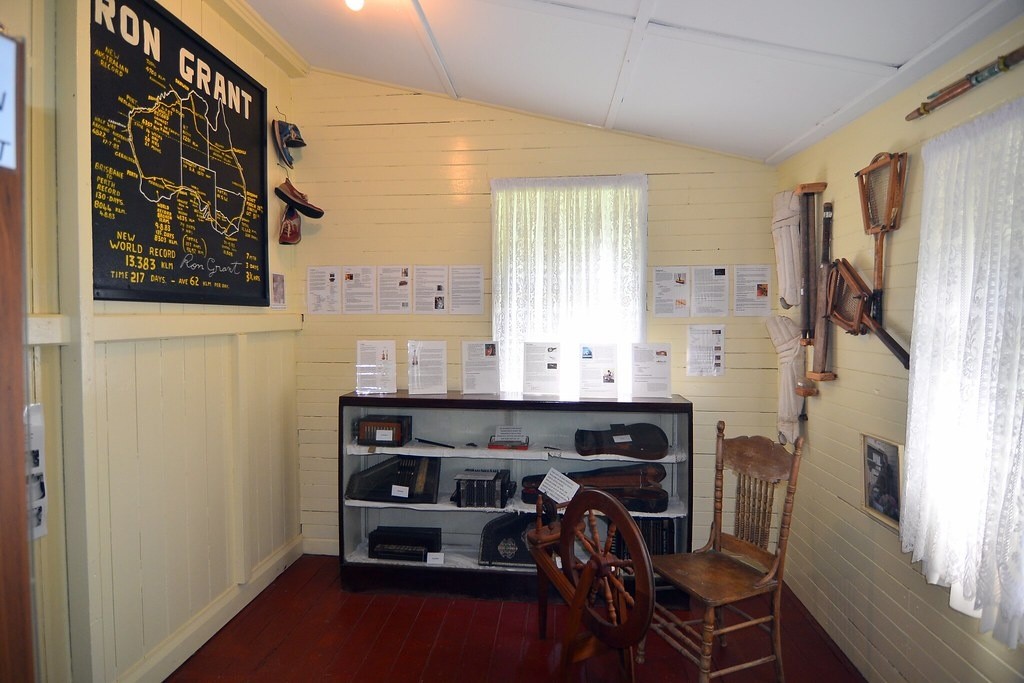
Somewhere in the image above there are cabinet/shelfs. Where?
[337,391,693,613]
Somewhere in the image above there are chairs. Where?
[636,421,804,683]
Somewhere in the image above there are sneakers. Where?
[273,119,293,169]
[279,205,302,244]
[286,122,306,146]
[274,178,324,218]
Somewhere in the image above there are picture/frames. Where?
[862,433,903,532]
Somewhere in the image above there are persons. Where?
[873,454,893,498]
